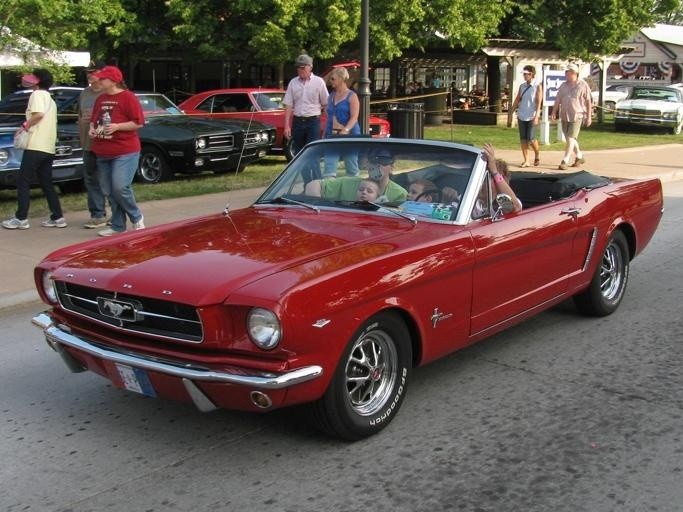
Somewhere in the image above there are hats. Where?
[82,63,104,73]
[92,66,123,83]
[20,69,53,88]
[562,63,578,74]
[520,68,535,74]
[294,54,313,69]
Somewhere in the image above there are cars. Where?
[591,85,632,113]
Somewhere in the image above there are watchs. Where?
[344,128,349,132]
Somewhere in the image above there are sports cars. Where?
[32,138,665,440]
[1,87,88,193]
[615,86,683,134]
[133,92,279,183]
[176,88,391,161]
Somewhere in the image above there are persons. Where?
[355,178,381,203]
[1,68,67,230]
[281,55,329,189]
[304,147,408,203]
[349,72,509,110]
[78,61,112,229]
[322,68,361,179]
[406,178,438,203]
[511,65,542,168]
[441,143,522,218]
[550,64,592,170]
[88,65,145,236]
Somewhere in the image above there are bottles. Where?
[103,112,112,139]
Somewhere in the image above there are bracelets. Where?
[491,173,497,177]
[494,175,503,183]
[21,121,29,130]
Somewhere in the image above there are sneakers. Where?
[106,216,113,226]
[83,217,106,230]
[0,215,31,231]
[97,228,127,236]
[520,161,530,168]
[571,158,586,168]
[130,214,145,231]
[557,160,569,170]
[41,217,68,229]
[533,156,540,167]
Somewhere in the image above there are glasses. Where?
[329,76,339,82]
[367,153,393,167]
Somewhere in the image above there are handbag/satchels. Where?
[12,127,32,150]
